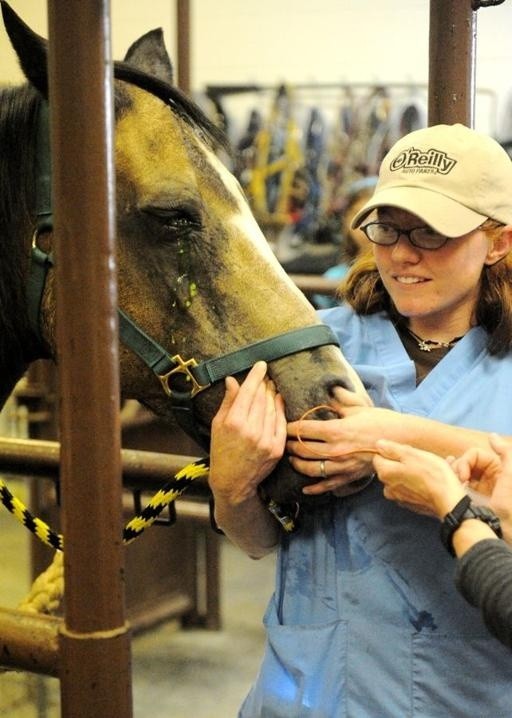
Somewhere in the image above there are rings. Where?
[318,457,330,479]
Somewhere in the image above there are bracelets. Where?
[439,493,504,559]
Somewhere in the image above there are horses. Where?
[0,1,376,522]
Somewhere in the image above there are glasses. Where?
[359,220,447,250]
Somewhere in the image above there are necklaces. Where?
[401,322,466,353]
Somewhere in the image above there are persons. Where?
[365,438,512,649]
[203,118,512,718]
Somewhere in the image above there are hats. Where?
[350,122,512,239]
